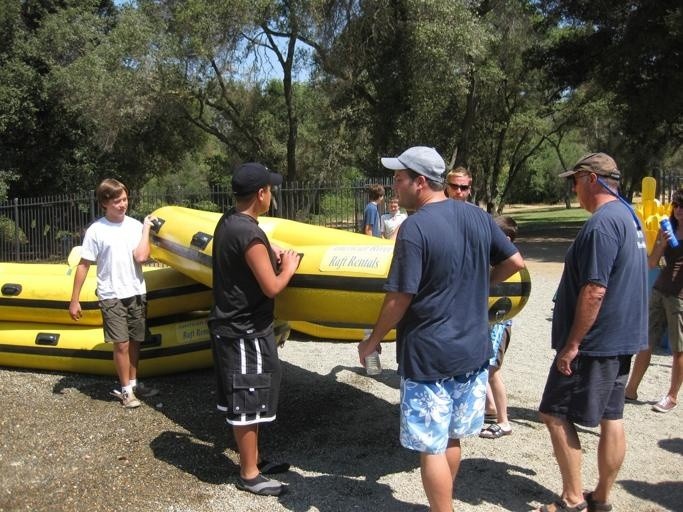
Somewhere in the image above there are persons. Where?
[69,177,159,410]
[622,190,683,413]
[380,195,408,241]
[445,166,473,203]
[480,214,526,439]
[356,145,526,511]
[361,184,386,239]
[204,162,302,496]
[528,153,649,512]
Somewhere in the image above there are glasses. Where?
[447,183,471,191]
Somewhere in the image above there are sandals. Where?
[484,410,497,423]
[529,499,589,512]
[479,422,512,438]
[582,489,613,512]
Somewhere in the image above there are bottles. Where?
[658,218,680,249]
[362,327,381,377]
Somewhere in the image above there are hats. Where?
[673,188,683,202]
[380,145,446,184]
[232,162,284,196]
[558,152,621,181]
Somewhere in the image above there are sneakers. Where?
[236,475,286,496]
[256,459,290,474]
[120,391,141,408]
[133,382,160,397]
[652,396,678,413]
[623,389,639,403]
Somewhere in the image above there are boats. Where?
[0,260,215,326]
[0,312,294,380]
[148,205,531,343]
